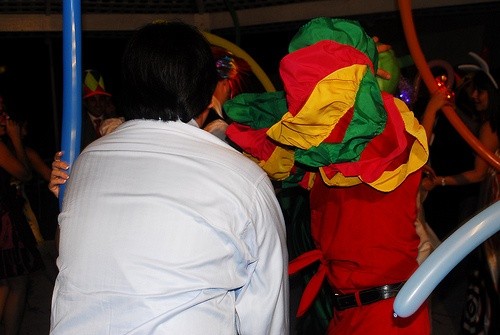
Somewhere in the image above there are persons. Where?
[0,0,500,335]
[49,20,291,334]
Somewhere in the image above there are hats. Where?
[83,69,112,99]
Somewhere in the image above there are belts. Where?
[329,284,409,310]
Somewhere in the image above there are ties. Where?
[93,119,102,135]
